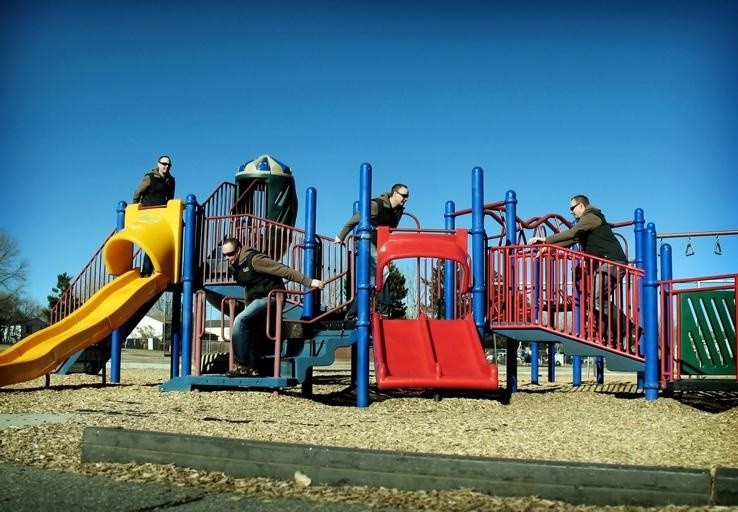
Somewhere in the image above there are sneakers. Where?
[344,313,358,321]
[225,361,261,378]
[623,326,640,352]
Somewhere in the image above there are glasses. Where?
[396,190,409,198]
[158,161,171,166]
[222,247,237,257]
[570,202,580,211]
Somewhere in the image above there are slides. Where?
[0,264,162,388]
[369,306,499,396]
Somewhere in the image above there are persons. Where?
[528,195,643,349]
[134,155,176,276]
[334,184,409,323]
[222,239,324,377]
